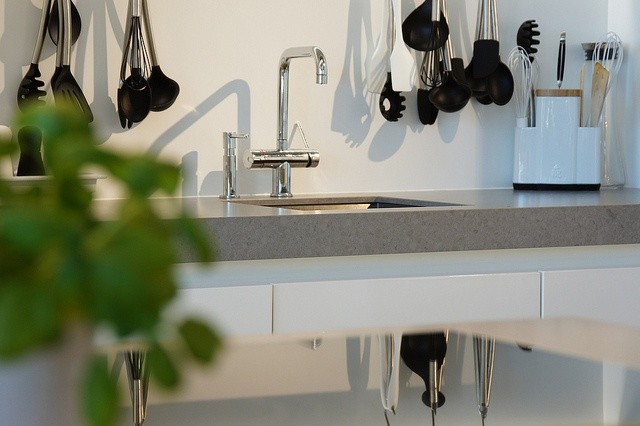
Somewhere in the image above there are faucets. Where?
[244,46,329,198]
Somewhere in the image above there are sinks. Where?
[222,195,468,212]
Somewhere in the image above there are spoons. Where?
[119,0,152,123]
[141,0,180,112]
[402,0,449,51]
[428,45,471,113]
[464,0,514,105]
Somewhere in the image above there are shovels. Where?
[591,61,609,126]
[51,0,94,124]
[390,0,416,92]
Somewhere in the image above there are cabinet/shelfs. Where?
[157,264,638,337]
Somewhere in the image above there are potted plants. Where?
[0,102,222,425]
